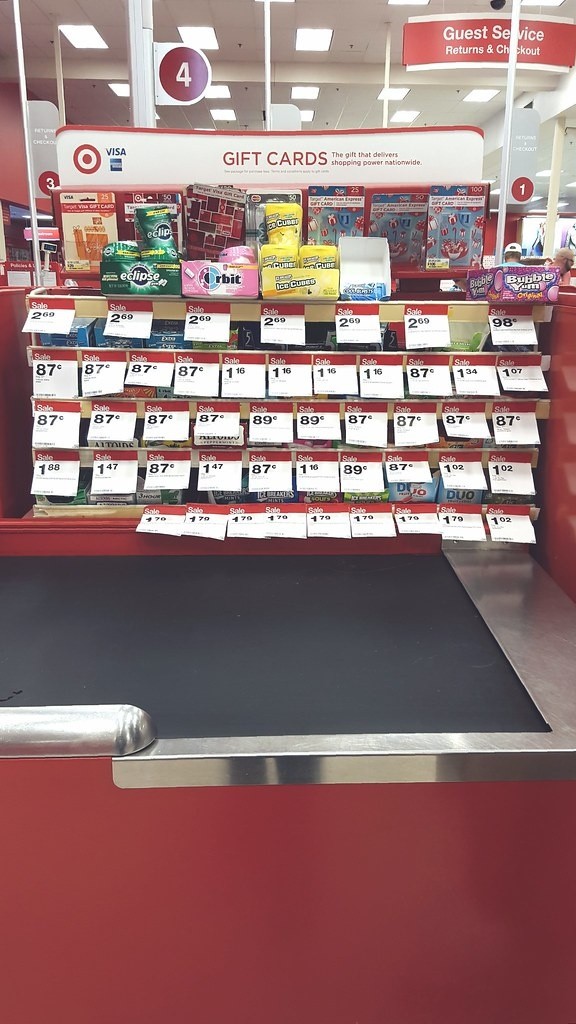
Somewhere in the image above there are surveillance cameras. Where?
[490,0,506,10]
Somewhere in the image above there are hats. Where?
[504,242,522,255]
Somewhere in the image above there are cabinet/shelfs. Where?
[24,122,552,553]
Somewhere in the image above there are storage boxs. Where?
[337,236,392,301]
[261,268,339,301]
[465,267,561,303]
[181,261,259,300]
[35,316,539,507]
[99,261,182,298]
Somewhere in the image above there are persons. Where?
[532,222,544,249]
[501,243,524,266]
[532,248,574,322]
[560,222,576,284]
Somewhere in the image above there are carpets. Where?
[3,551,552,740]
